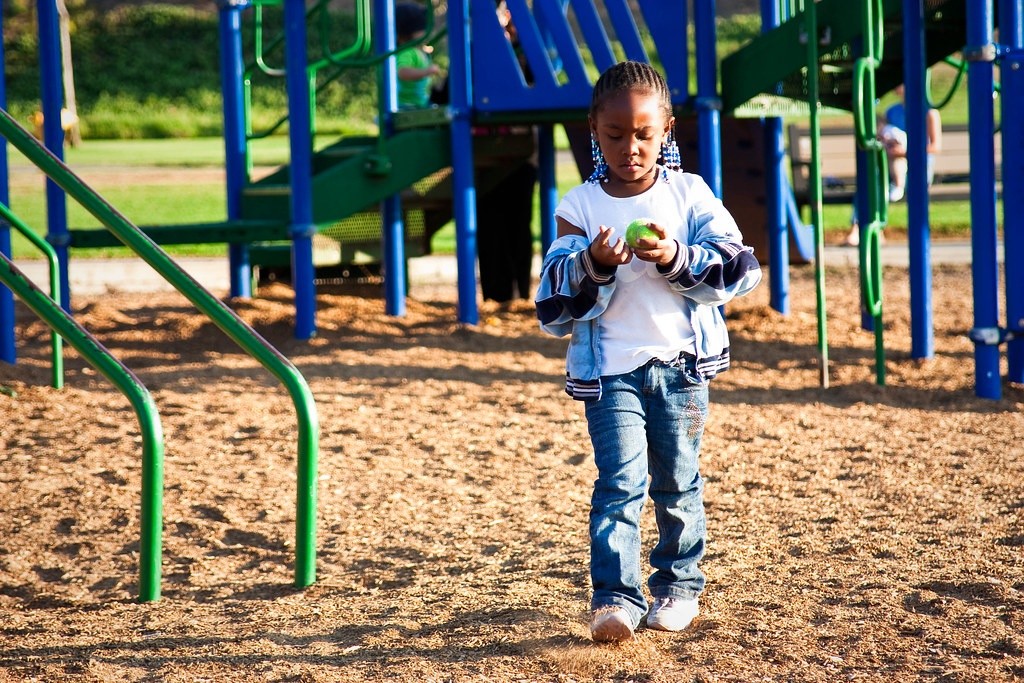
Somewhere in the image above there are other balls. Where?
[625,218,660,249]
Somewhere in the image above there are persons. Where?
[531,61,764,645]
[875,111,907,204]
[392,0,443,114]
[470,1,542,310]
[846,82,943,247]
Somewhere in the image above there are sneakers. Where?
[646,597,699,632]
[591,606,634,640]
